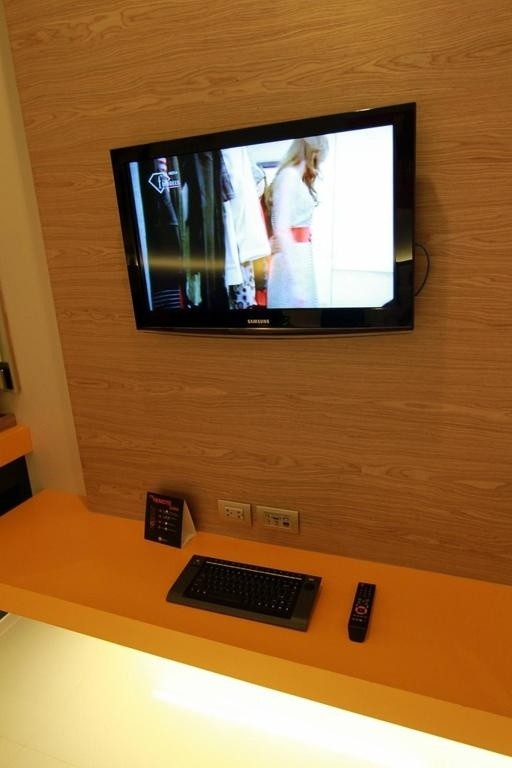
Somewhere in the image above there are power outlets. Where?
[217,500,252,527]
[256,506,299,534]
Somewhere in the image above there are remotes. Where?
[346,581,377,643]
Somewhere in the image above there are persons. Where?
[265,136,328,309]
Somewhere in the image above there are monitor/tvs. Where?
[109,100,417,339]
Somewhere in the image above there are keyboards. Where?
[167,553,322,632]
